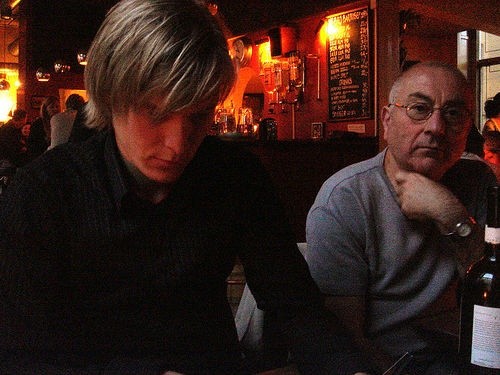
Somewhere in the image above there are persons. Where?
[481,92,500,185]
[306,61,500,375]
[0,94,108,169]
[0,0,368,375]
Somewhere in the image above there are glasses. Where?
[387,102,477,125]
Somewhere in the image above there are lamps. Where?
[0,72,10,92]
[77,47,90,66]
[35,66,50,83]
[1,4,13,20]
[54,58,72,75]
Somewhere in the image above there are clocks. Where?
[231,37,252,66]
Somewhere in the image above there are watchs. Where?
[441,214,476,242]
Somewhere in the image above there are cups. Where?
[214,108,253,136]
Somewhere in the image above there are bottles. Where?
[456,186,500,375]
[264,64,276,104]
[274,61,290,103]
[290,51,303,98]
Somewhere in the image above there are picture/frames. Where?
[311,122,324,138]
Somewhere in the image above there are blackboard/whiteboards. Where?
[324,6,372,121]
